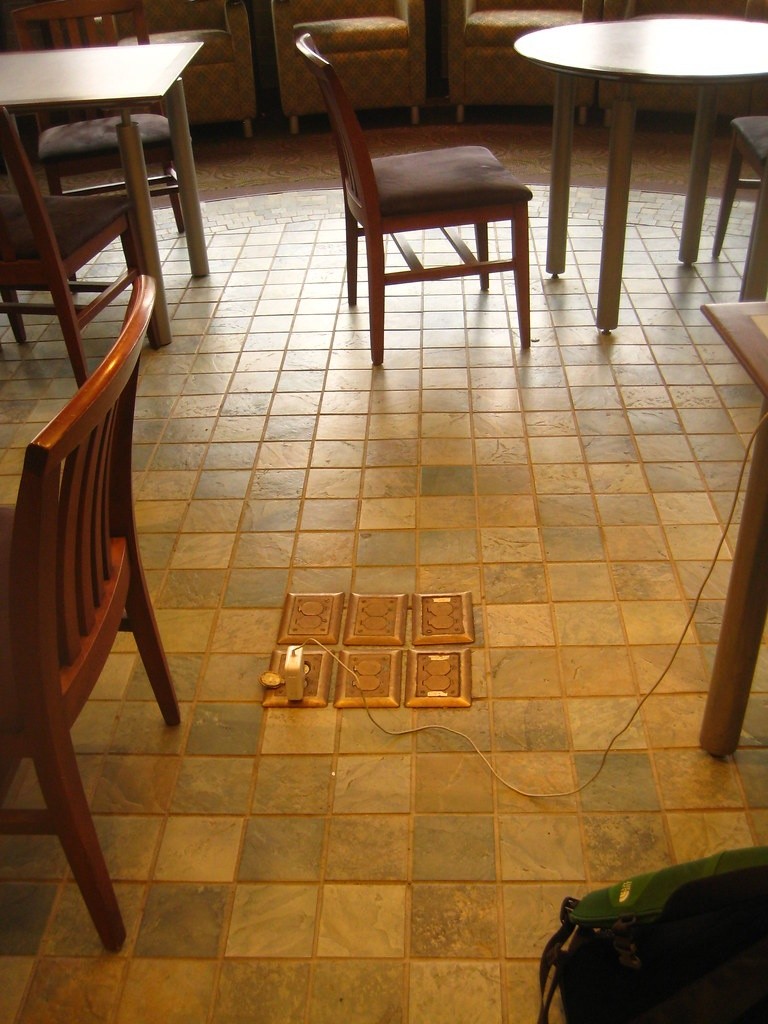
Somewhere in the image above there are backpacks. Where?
[538,845,768,1024]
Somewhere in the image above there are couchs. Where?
[441,0,600,124]
[600,0,768,129]
[270,0,426,136]
[94,0,257,139]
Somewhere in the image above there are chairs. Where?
[296,33,534,365]
[713,116,768,258]
[0,106,160,390]
[11,0,185,293]
[0,277,180,954]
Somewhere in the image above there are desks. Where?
[514,18,768,335]
[0,42,205,347]
[699,304,768,759]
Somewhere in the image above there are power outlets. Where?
[276,592,344,645]
[335,650,403,708]
[343,592,407,646]
[404,649,472,707]
[262,648,335,709]
[412,591,474,646]
